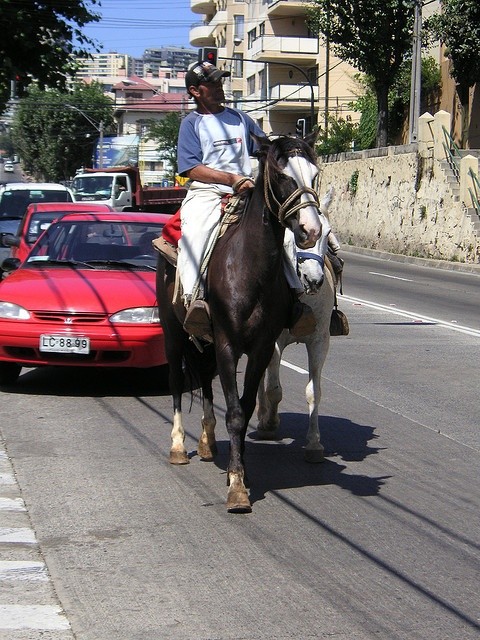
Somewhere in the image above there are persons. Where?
[172,60,269,312]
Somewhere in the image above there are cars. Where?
[1,212,187,391]
[4,164,14,172]
[0,183,75,260]
[2,203,132,264]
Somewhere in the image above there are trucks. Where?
[71,166,188,214]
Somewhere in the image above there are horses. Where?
[257,186,350,464]
[150,125,324,514]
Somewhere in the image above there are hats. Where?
[185,61,231,99]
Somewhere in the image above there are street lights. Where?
[64,104,104,170]
[122,79,186,118]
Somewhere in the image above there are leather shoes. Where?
[182,300,211,339]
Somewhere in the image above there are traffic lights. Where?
[198,47,217,65]
[296,118,308,140]
